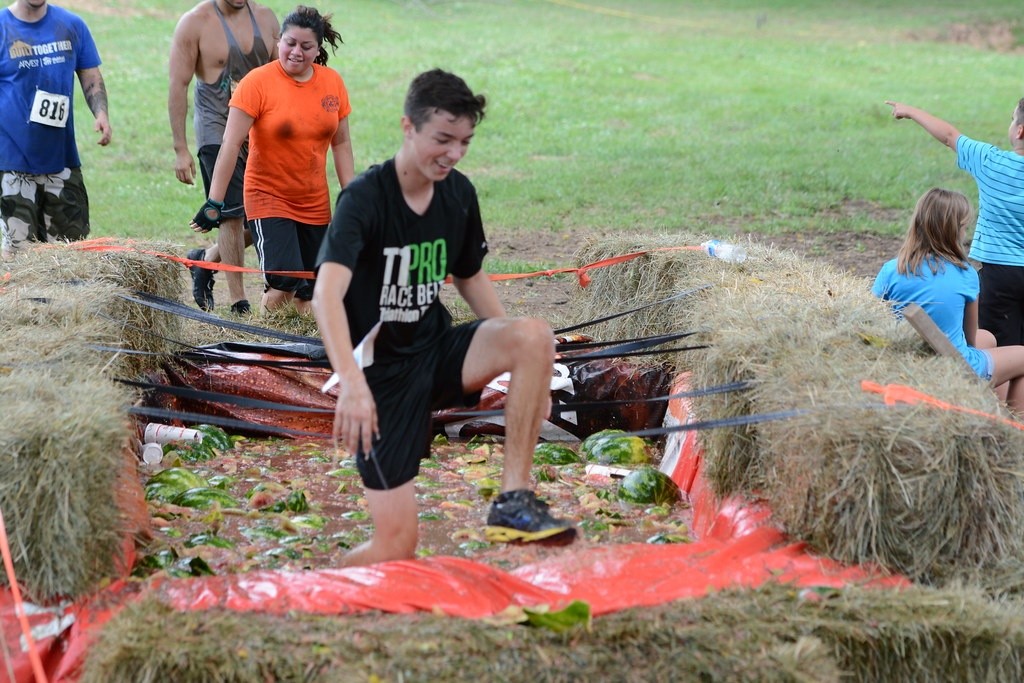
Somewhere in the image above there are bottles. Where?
[701,239,746,264]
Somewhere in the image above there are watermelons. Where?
[530,425,683,506]
[139,424,307,514]
[183,534,234,549]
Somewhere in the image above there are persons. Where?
[314,68,577,566]
[189,5,355,321]
[168,0,281,317]
[0,0,112,258]
[885,97,1024,403]
[871,187,1024,422]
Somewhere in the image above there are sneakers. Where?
[230,299,254,319]
[488,491,576,546]
[186,251,220,311]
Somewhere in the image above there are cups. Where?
[140,442,164,464]
[144,422,202,445]
